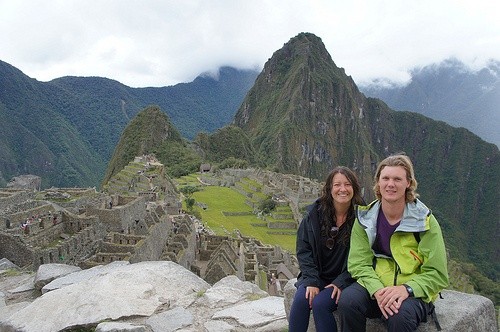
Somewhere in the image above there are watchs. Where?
[401,284,414,298]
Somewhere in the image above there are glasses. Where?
[326,227,338,249]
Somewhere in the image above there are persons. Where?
[286,166,368,332]
[338,155,450,332]
